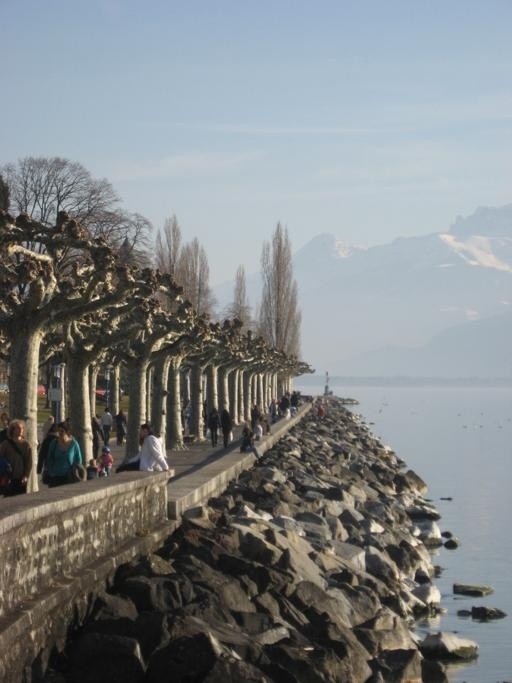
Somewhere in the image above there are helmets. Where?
[102,447,110,453]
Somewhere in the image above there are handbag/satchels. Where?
[121,423,127,435]
[229,431,233,442]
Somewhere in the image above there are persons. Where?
[203,391,303,462]
[181,399,193,437]
[318,397,328,423]
[0,407,168,498]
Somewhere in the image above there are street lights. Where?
[103,364,114,410]
[51,363,62,426]
